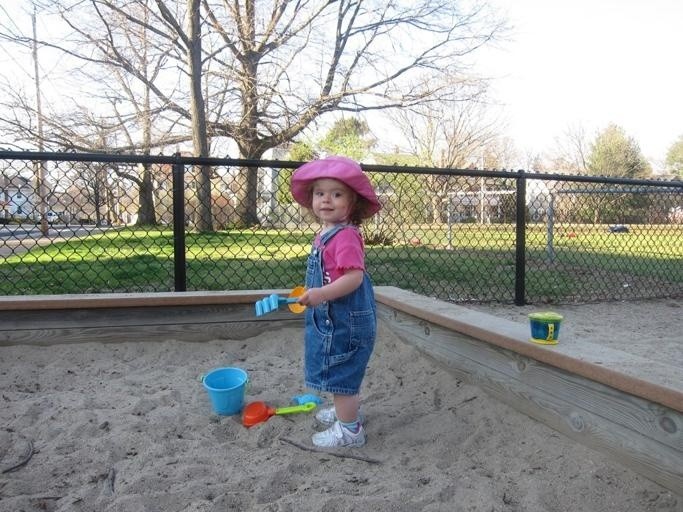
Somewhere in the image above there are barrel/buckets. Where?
[202,365,247,415]
[528,312,564,345]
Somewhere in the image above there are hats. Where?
[290,155,381,219]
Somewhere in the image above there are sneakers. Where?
[315,407,364,428]
[311,420,366,449]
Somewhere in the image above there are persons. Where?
[289,155,379,446]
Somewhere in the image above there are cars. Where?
[36,211,59,224]
